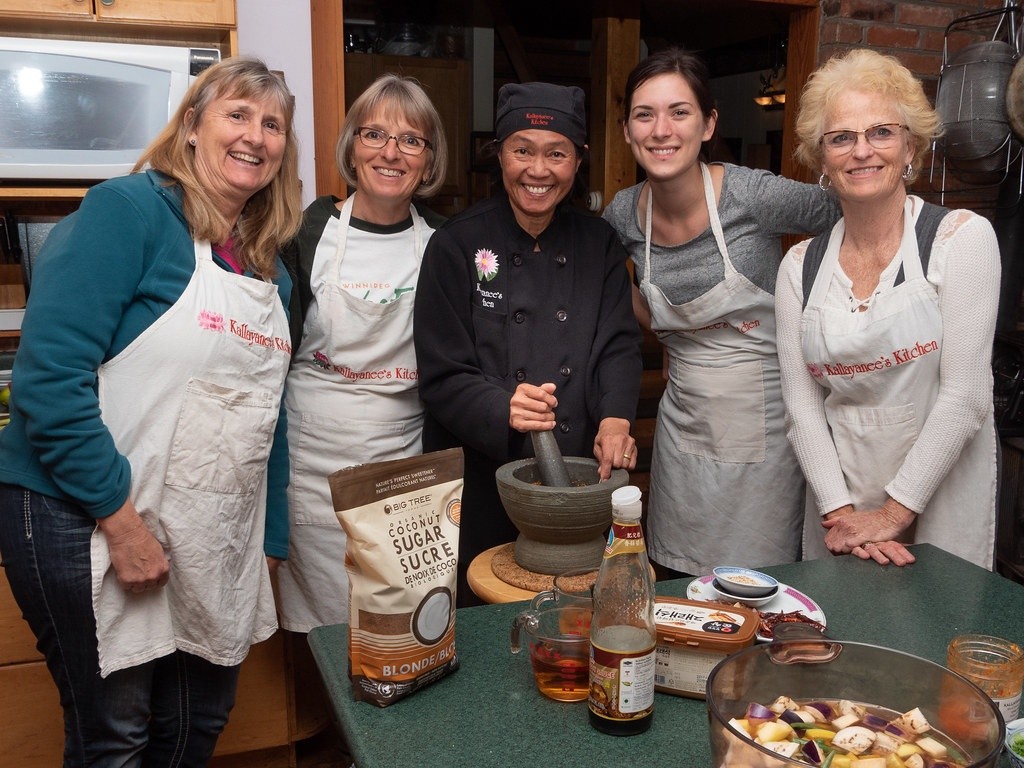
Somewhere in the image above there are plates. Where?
[686,575,827,642]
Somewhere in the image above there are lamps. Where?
[753,89,785,111]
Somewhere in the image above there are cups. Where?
[510,607,593,702]
[531,566,599,616]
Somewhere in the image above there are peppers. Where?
[757,609,829,637]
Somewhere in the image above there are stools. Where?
[466,545,657,603]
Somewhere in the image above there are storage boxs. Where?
[652,593,760,703]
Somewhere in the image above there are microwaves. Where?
[0,37,222,179]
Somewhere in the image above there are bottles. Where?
[947,633,1024,725]
[344,25,387,54]
[588,486,655,736]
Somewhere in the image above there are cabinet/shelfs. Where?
[0,0,300,768]
[346,51,473,201]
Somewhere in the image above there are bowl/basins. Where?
[1004,718,1024,768]
[713,567,779,608]
[706,640,1006,768]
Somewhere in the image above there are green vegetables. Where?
[1010,733,1024,760]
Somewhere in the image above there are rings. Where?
[623,455,631,460]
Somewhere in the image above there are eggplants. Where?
[743,700,908,766]
[924,760,952,768]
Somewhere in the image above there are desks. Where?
[304,542,1024,768]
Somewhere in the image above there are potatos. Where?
[732,718,925,768]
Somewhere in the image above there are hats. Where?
[494,82,589,147]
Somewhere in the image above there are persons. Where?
[276,75,447,706]
[774,49,1001,577]
[595,50,843,581]
[414,81,644,617]
[0,57,301,768]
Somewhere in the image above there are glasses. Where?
[353,126,432,156]
[819,124,909,157]
